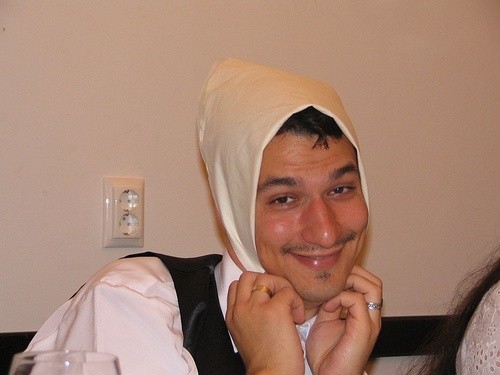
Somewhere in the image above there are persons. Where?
[402,247,500,374]
[14,56,384,373]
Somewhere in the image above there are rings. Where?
[251,285,272,297]
[366,299,383,310]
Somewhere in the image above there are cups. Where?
[9,350,120,375]
